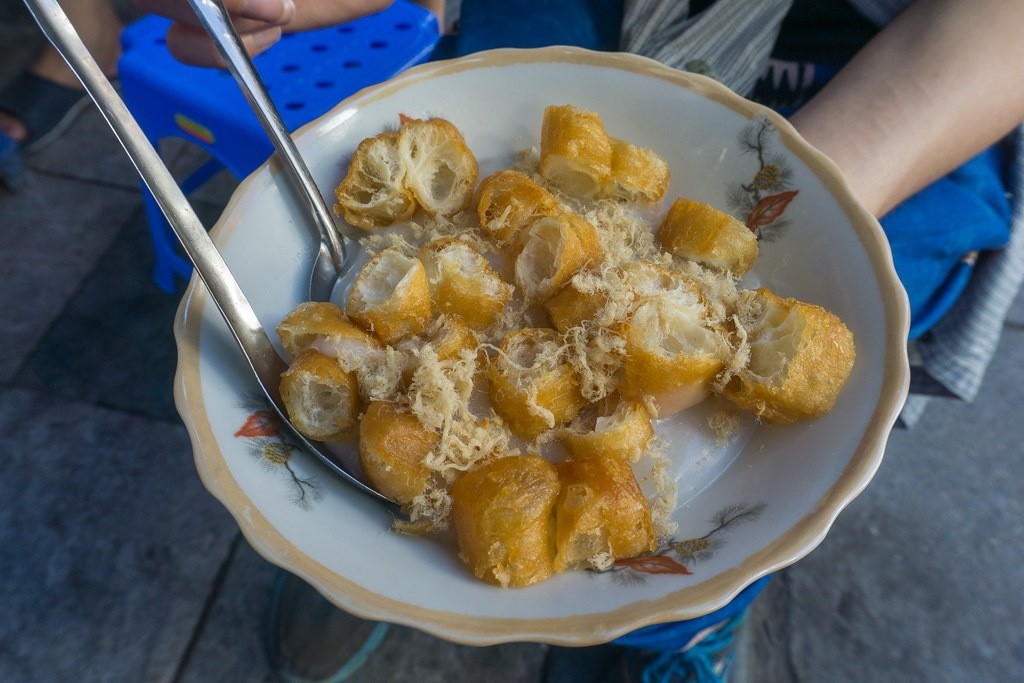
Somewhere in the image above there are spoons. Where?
[22,0,402,508]
[187,0,370,302]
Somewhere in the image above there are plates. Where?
[169,42,912,647]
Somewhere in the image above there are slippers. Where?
[0,38,121,156]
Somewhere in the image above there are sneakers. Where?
[621,613,742,682]
[262,569,390,682]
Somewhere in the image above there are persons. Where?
[0,0,135,161]
[133,0,1023,682]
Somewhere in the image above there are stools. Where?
[117,0,443,295]
